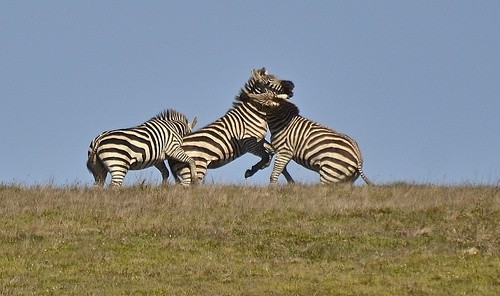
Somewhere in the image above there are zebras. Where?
[167,67,373,186]
[85,108,198,189]
